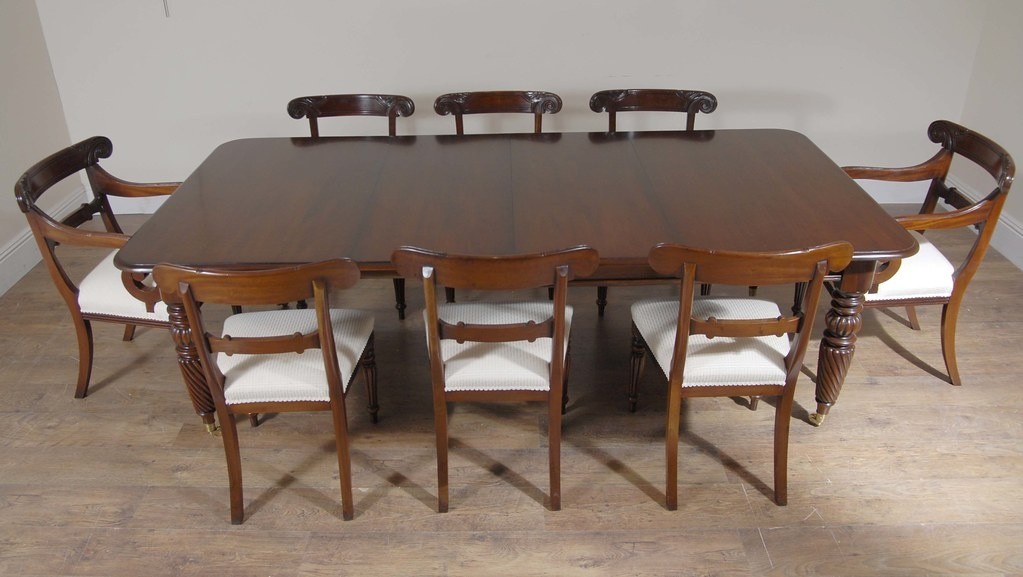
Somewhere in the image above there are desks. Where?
[112,128,920,436]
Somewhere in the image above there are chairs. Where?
[14,134,243,399]
[151,258,380,526]
[388,244,601,512]
[288,94,415,320]
[791,119,1014,386]
[627,241,854,510]
[589,89,717,317]
[434,91,563,302]
